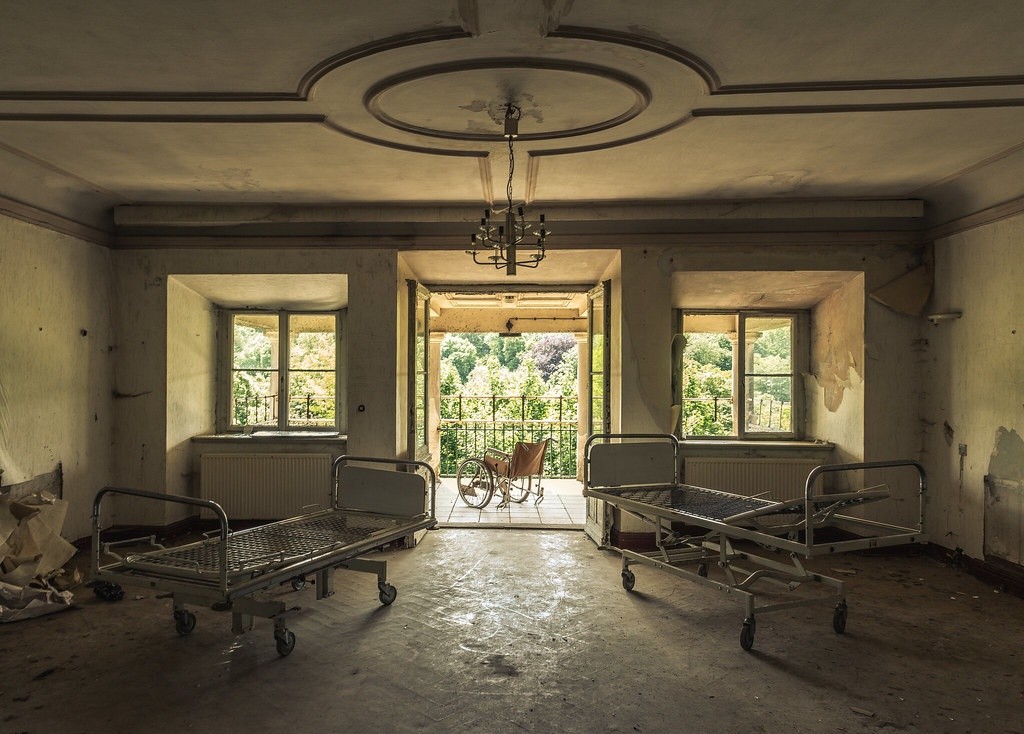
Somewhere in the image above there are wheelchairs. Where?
[455,438,558,509]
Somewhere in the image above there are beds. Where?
[582,433,927,651]
[90,455,438,656]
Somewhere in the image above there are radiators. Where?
[685,457,824,526]
[199,453,334,519]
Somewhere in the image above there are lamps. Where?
[466,102,553,273]
